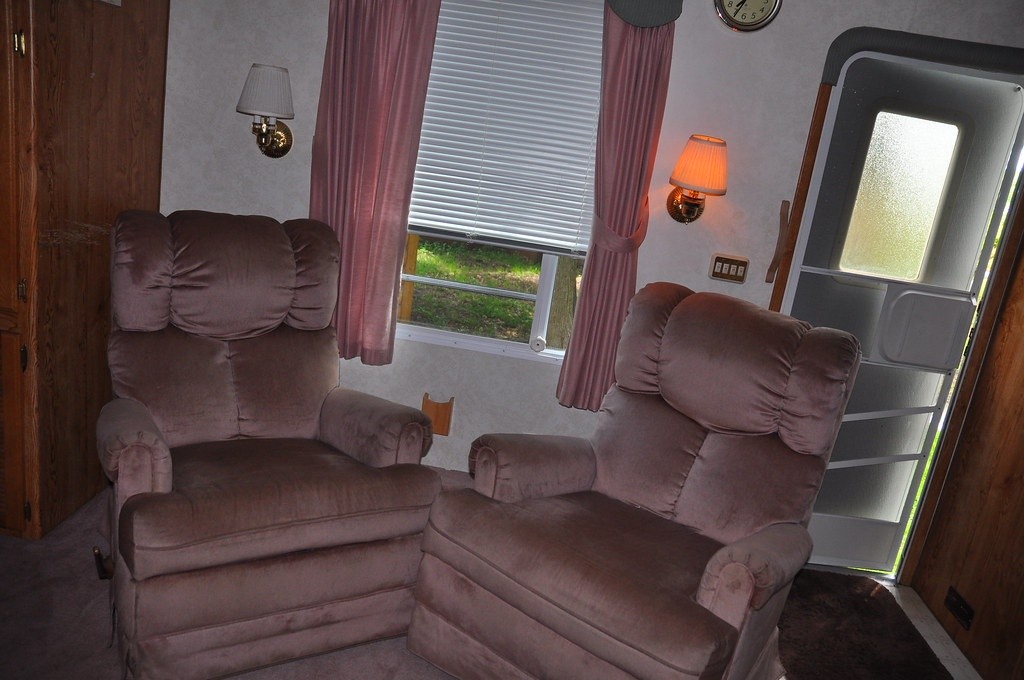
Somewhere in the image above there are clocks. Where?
[714,0,782,31]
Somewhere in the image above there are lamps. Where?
[236,62,294,158]
[666,135,727,224]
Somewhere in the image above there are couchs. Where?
[409,282,863,680]
[92,211,440,680]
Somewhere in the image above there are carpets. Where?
[776,569,954,680]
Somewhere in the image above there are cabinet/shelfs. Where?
[0,0,170,544]
